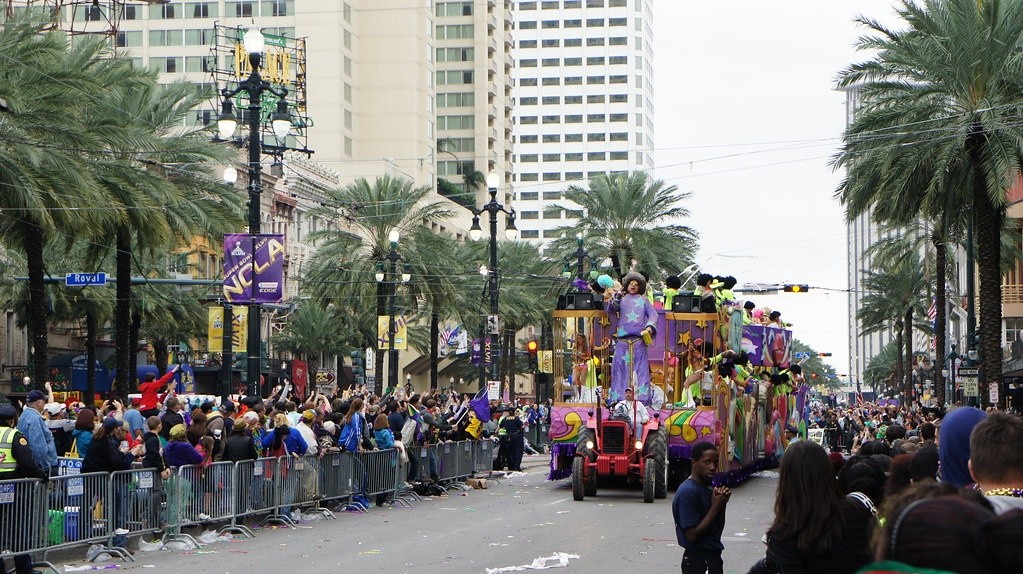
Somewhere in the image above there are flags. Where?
[857,378,864,405]
[470,390,491,423]
[928,294,955,322]
[438,326,452,345]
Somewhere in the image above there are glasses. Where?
[70,403,79,407]
[120,428,129,434]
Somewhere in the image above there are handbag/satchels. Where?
[64,437,79,458]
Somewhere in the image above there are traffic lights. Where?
[528,341,537,370]
[785,284,810,293]
[819,353,832,358]
[813,374,820,376]
[235,352,245,370]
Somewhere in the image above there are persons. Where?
[671,441,733,574]
[607,270,659,407]
[744,398,1022,573]
[1,366,548,574]
[615,388,649,450]
[568,273,806,407]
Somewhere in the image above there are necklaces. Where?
[625,400,633,410]
[985,488,1023,499]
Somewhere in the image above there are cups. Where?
[214,429,222,437]
[121,440,129,452]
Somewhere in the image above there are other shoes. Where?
[514,467,522,472]
[409,480,420,485]
[29,569,42,574]
[113,528,129,536]
[285,520,299,525]
[381,502,389,507]
[198,513,210,520]
[403,481,413,488]
[321,509,332,516]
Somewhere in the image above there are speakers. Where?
[674,295,702,313]
[702,293,717,313]
[557,293,605,310]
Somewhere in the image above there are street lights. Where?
[374,230,411,391]
[941,335,967,407]
[468,172,518,379]
[560,228,600,289]
[214,24,294,400]
[877,360,929,400]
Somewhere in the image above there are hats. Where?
[103,416,123,426]
[232,418,249,431]
[302,409,317,420]
[220,401,236,413]
[369,405,379,416]
[25,390,49,403]
[0,406,17,419]
[624,387,634,393]
[64,397,78,406]
[48,401,66,416]
[131,397,141,404]
[169,424,186,437]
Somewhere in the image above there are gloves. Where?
[35,470,51,485]
